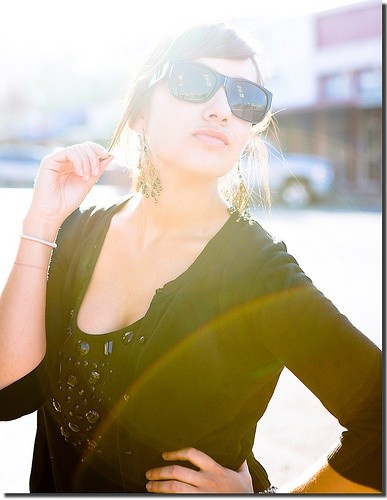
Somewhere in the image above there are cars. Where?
[220,138,333,208]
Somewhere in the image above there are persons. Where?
[0,21,382,493]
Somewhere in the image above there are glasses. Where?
[136,60,273,124]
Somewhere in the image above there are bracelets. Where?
[14,263,47,272]
[21,234,58,249]
